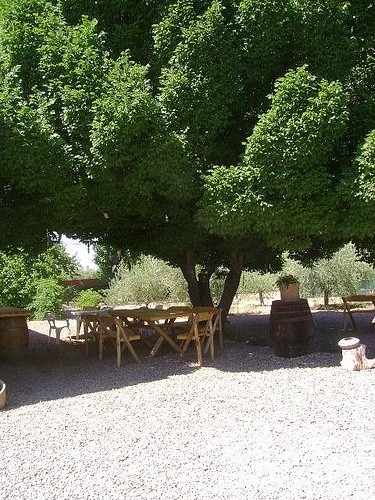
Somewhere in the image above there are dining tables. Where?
[105,309,191,359]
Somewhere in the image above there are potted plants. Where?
[273,275,300,302]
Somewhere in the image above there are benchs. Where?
[342,295,375,331]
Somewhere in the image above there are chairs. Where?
[45,311,71,344]
[165,306,192,349]
[192,307,223,353]
[97,316,141,366]
[177,311,214,363]
